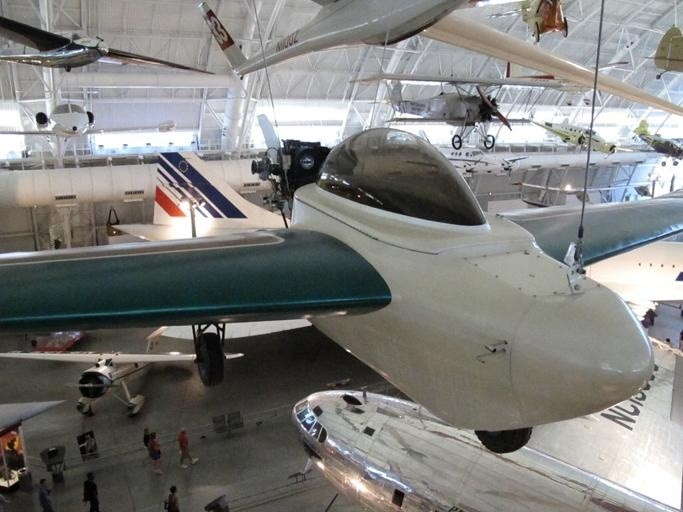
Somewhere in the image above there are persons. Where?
[179,429,199,468]
[38,477,55,512]
[640,315,650,328]
[678,329,682,352]
[147,431,165,473]
[645,308,657,326]
[83,471,100,510]
[666,338,673,348]
[165,485,180,511]
[142,425,151,464]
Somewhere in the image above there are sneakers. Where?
[153,456,200,476]
